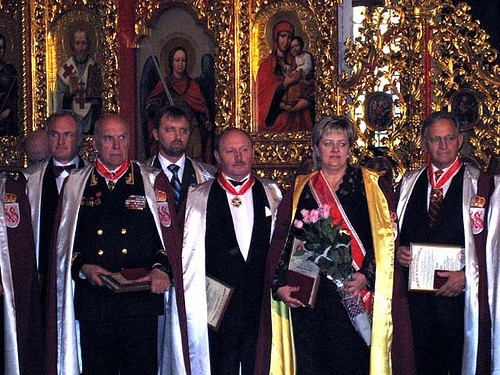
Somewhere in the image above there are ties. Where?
[428,170,444,232]
[167,164,183,216]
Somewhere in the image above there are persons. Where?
[179,126,283,375]
[396,111,500,375]
[253,115,417,375]
[0,106,219,375]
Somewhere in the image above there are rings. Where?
[451,293,454,297]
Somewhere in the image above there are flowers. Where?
[293,204,371,347]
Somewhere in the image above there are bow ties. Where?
[53,163,76,178]
[228,179,248,187]
